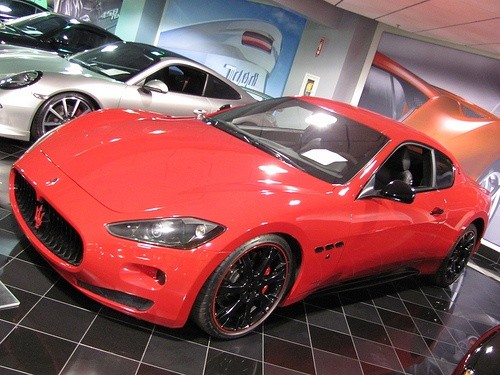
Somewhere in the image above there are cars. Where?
[357,52,500,228]
[0,41,260,147]
[8,95,491,338]
[0,12,127,58]
[0,0,51,30]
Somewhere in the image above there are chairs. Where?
[157,70,192,93]
[143,68,166,85]
[291,121,353,172]
[372,144,415,193]
